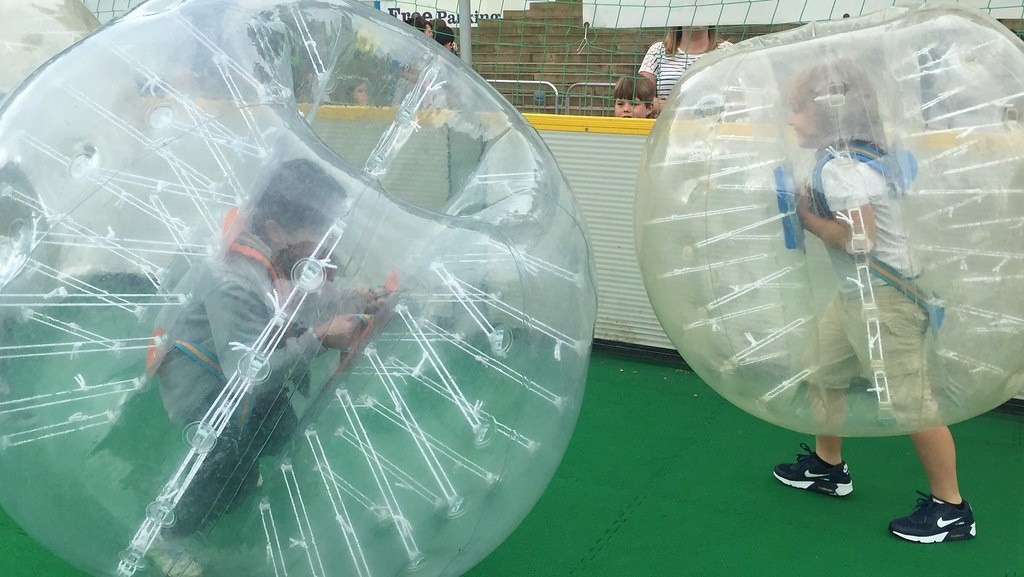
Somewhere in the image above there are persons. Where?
[613,73,655,118]
[342,76,369,106]
[428,26,459,107]
[637,24,755,119]
[771,59,981,543]
[389,17,433,105]
[230,26,331,103]
[428,18,458,56]
[138,155,391,577]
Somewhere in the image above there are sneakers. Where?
[771,453,855,496]
[888,490,977,544]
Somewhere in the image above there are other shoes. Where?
[150,546,203,577]
